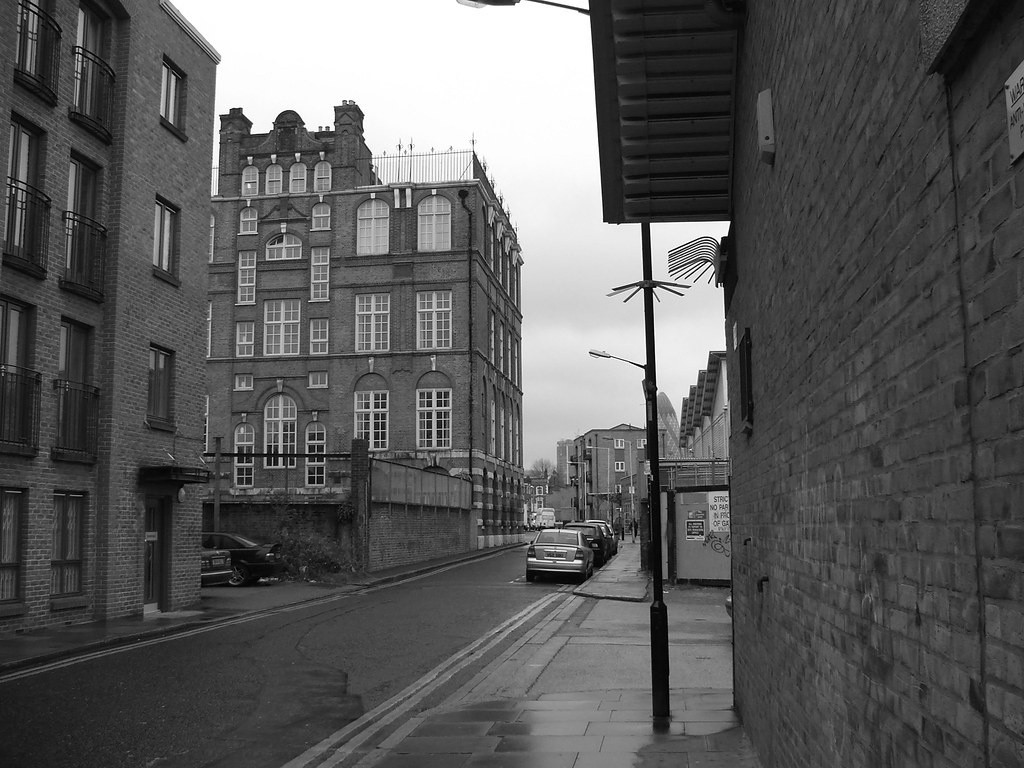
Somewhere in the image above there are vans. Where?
[529,507,556,528]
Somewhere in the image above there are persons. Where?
[629,518,638,538]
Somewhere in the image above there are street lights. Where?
[455,0,673,732]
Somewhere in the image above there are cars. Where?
[199,531,288,588]
[526,519,619,584]
